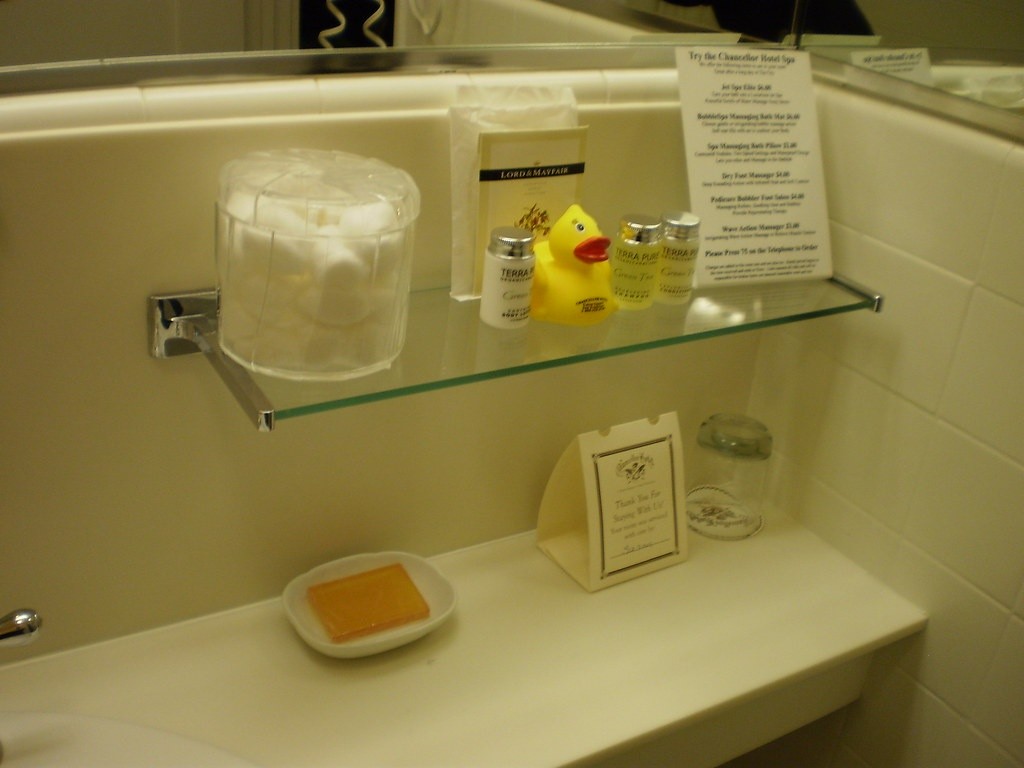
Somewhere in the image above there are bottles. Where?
[475,225,540,330]
[657,210,701,305]
[608,211,670,311]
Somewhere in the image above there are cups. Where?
[680,412,770,543]
[211,149,425,382]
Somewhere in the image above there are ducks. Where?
[531,205,617,326]
